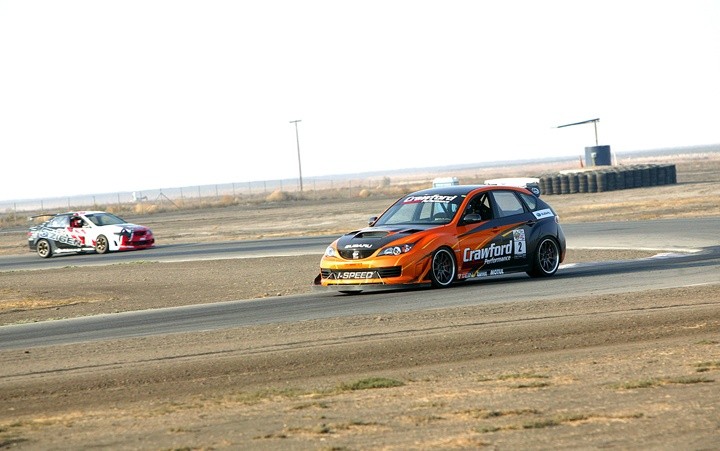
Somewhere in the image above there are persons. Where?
[70,217,79,227]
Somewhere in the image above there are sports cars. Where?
[27,210,155,258]
[313,185,567,295]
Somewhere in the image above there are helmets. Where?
[448,197,474,219]
[73,218,82,227]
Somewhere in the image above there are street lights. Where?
[289,119,303,191]
